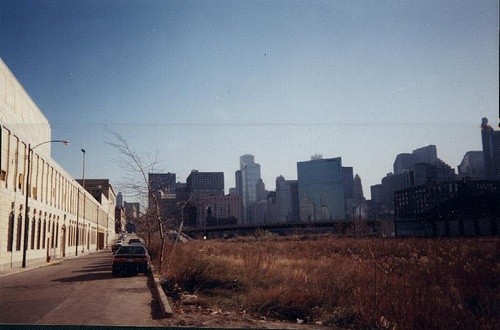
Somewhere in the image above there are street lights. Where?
[75,184,101,256]
[80,147,86,188]
[22,139,68,268]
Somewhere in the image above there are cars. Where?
[111,244,151,277]
[112,231,141,255]
